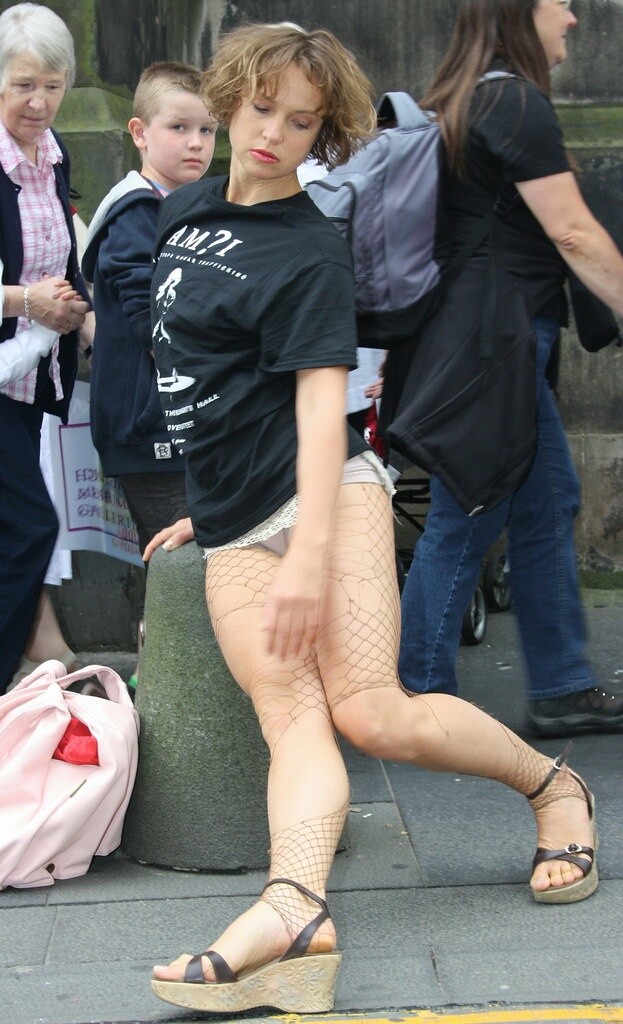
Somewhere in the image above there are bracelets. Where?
[24,284,32,326]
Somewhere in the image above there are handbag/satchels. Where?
[48,378,160,570]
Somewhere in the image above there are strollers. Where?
[362,424,513,647]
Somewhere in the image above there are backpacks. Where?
[304,69,520,347]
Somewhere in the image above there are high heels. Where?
[148,876,344,1013]
[525,753,599,905]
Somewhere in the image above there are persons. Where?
[142,0,623,1013]
[0,0,221,695]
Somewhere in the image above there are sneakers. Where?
[526,687,623,737]
